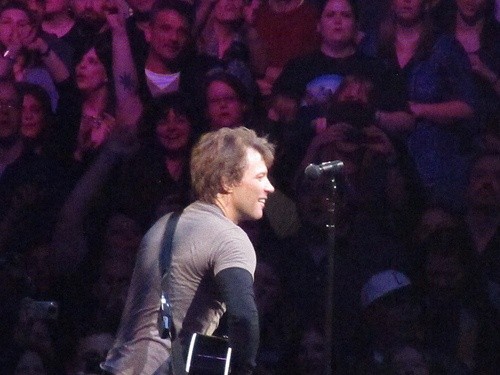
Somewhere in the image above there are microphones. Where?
[304,161,344,180]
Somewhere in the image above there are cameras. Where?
[28,302,58,320]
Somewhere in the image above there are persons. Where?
[99,126,278,375]
[201,0,500,375]
[0,0,223,375]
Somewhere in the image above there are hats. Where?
[361,270,412,312]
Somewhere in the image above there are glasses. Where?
[0,103,21,113]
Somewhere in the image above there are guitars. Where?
[185,331,233,375]
